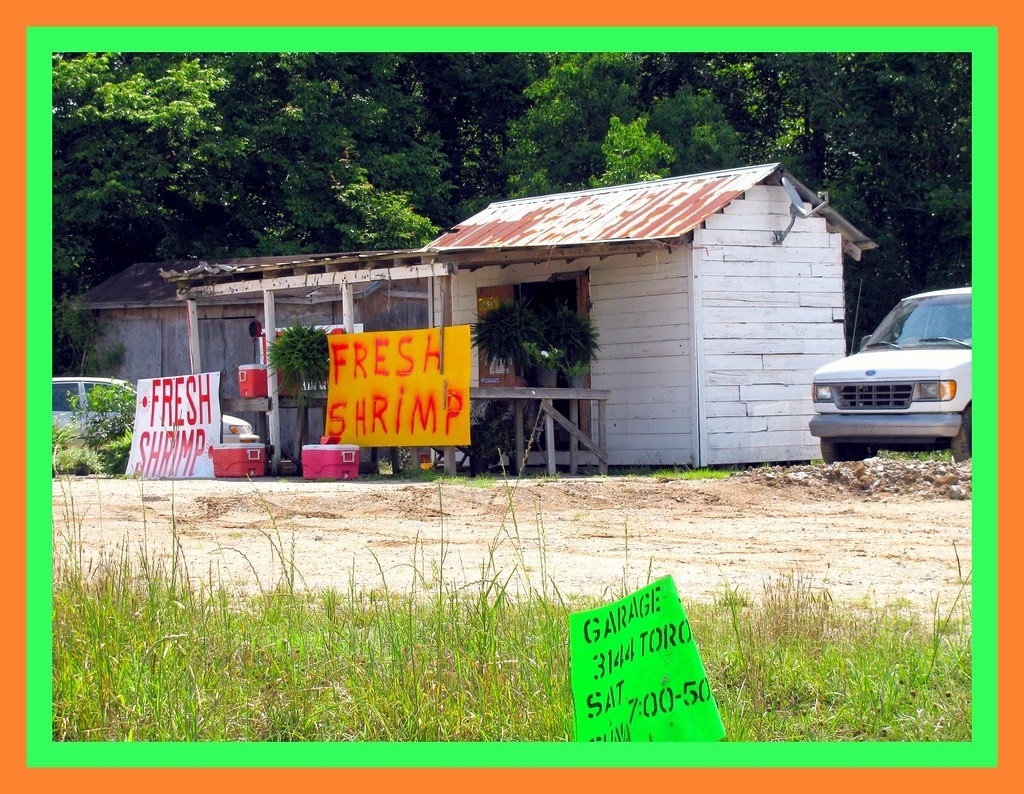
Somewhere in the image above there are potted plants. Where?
[563,361,590,388]
[501,410,531,477]
[263,316,328,398]
[464,412,508,478]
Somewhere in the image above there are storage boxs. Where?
[239,364,300,398]
[213,443,266,477]
[302,444,361,481]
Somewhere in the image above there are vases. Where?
[529,368,559,388]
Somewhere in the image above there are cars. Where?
[52,377,260,442]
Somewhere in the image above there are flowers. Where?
[522,342,567,371]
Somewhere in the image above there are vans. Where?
[808,287,973,464]
